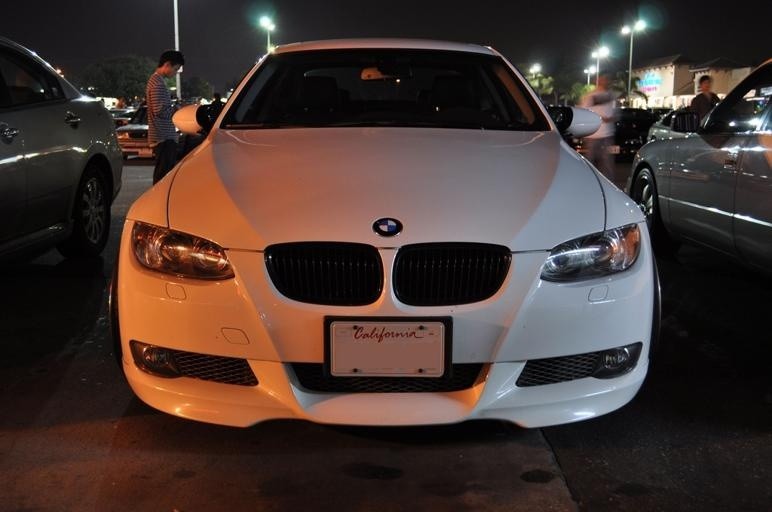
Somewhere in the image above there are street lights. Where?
[169,87,177,98]
[591,47,609,88]
[621,20,648,109]
[583,65,597,87]
[529,63,544,101]
[259,16,275,54]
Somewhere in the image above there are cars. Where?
[104,96,209,160]
[615,56,772,272]
[112,36,661,440]
[2,40,125,276]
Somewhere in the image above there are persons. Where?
[579,71,620,184]
[144,49,186,187]
[690,74,720,123]
[116,95,125,109]
